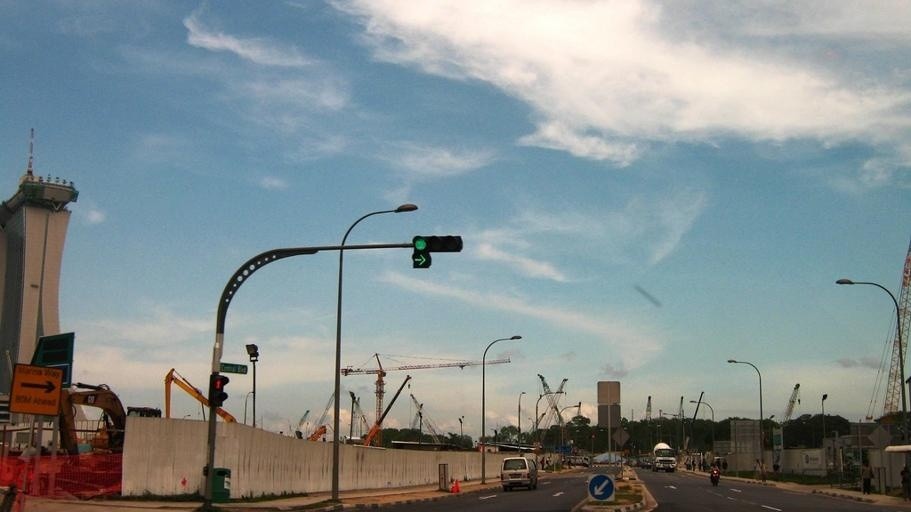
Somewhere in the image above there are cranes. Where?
[341,353,511,446]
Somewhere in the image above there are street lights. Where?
[822,394,827,448]
[459,416,464,437]
[690,401,714,440]
[835,279,907,445]
[518,392,526,451]
[495,430,497,452]
[728,360,762,460]
[327,204,419,503]
[480,335,522,485]
[663,413,685,450]
[244,344,259,427]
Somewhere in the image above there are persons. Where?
[540,457,551,470]
[754,459,762,481]
[900,465,911,500]
[861,459,875,494]
[686,457,727,481]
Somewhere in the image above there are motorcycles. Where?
[709,470,721,487]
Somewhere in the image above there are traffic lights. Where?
[413,236,463,252]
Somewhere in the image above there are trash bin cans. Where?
[210,468,231,503]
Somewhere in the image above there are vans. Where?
[501,457,537,491]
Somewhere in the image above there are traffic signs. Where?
[8,363,64,416]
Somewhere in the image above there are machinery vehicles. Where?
[160,369,442,450]
[59,383,161,479]
[645,396,652,422]
[865,239,911,422]
[527,374,582,446]
[653,443,677,472]
[777,383,800,424]
